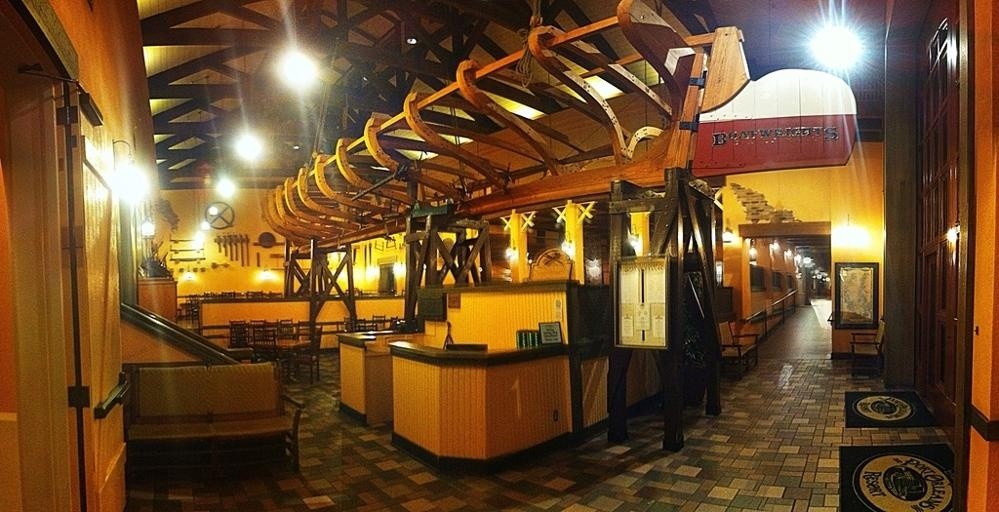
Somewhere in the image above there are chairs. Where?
[186,290,399,384]
[446,344,487,351]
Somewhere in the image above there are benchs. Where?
[123,361,306,469]
[850,319,885,379]
[719,320,760,381]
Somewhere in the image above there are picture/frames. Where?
[539,322,563,345]
[835,261,879,329]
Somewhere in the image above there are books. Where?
[522,331,527,348]
[527,331,530,348]
[534,331,540,348]
[528,332,535,349]
[515,329,522,349]
[445,342,489,351]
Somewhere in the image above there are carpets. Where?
[839,442,964,512]
[845,390,939,428]
